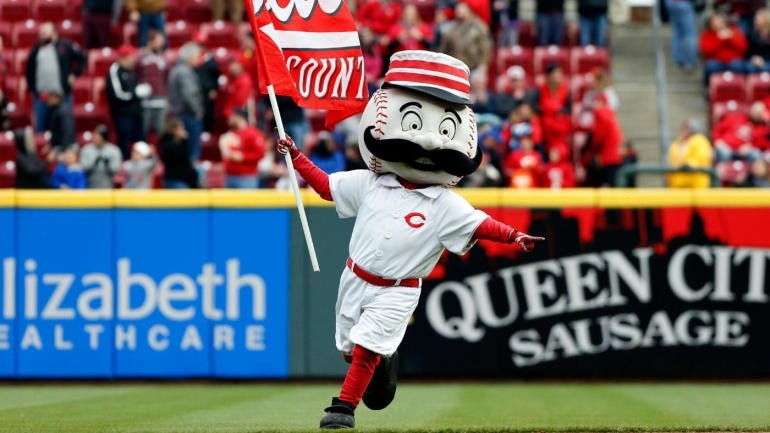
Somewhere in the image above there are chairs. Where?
[0,0,770,189]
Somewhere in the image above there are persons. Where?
[50,142,87,189]
[657,0,706,73]
[665,119,715,188]
[122,142,159,188]
[15,125,60,190]
[81,124,122,189]
[82,0,623,188]
[278,50,545,428]
[699,9,770,87]
[0,33,11,122]
[712,102,770,183]
[22,22,88,152]
[106,42,145,161]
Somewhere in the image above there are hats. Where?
[593,90,606,101]
[381,50,474,106]
[118,43,139,56]
[233,106,249,121]
[135,142,151,155]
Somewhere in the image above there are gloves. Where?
[274,127,300,159]
[511,229,546,251]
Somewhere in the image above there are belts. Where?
[346,257,423,288]
